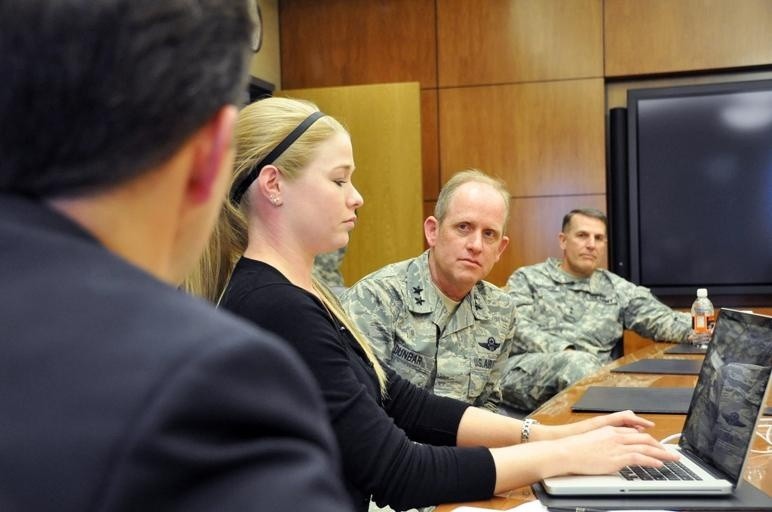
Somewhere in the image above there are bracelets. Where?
[515,416,540,446]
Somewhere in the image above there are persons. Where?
[346,168,520,415]
[504,203,695,407]
[184,92,686,509]
[1,2,359,509]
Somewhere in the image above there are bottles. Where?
[690,288,716,350]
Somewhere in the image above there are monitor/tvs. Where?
[627,78,772,295]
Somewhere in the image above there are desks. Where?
[436,341,772,512]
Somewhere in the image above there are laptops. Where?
[539,308,772,496]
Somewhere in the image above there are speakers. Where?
[610,107,630,282]
